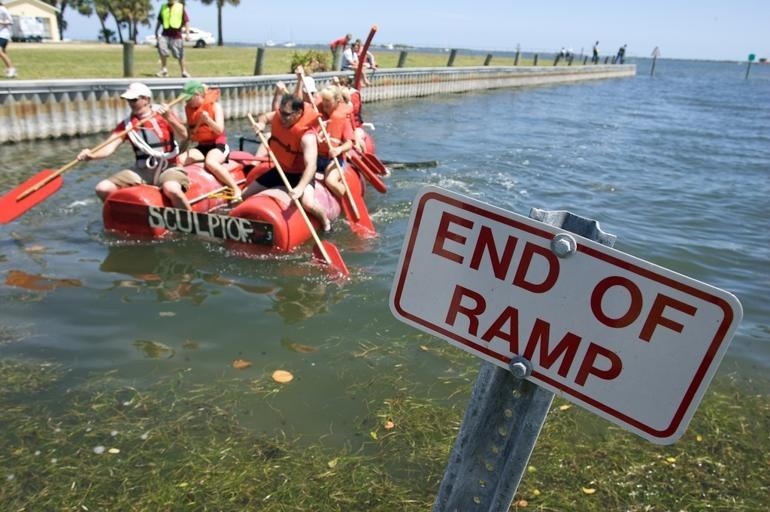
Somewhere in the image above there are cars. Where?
[146,27,216,47]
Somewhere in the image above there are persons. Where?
[77,81,194,212]
[0,1,16,78]
[329,33,353,73]
[241,92,334,233]
[332,75,368,153]
[241,76,318,178]
[154,0,191,79]
[294,63,357,199]
[355,37,378,70]
[178,78,243,205]
[341,42,372,89]
[613,43,627,64]
[592,41,600,65]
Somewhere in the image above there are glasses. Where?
[277,109,295,116]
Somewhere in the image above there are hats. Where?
[119,82,155,100]
[176,80,206,101]
[300,77,317,96]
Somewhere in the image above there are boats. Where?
[266,41,277,47]
[282,41,297,48]
[103,129,376,254]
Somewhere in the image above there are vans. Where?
[11,15,45,42]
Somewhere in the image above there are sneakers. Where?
[155,69,192,79]
[6,68,17,78]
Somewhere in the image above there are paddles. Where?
[0,95,189,225]
[352,144,387,176]
[296,66,377,239]
[245,112,350,283]
[344,147,388,195]
